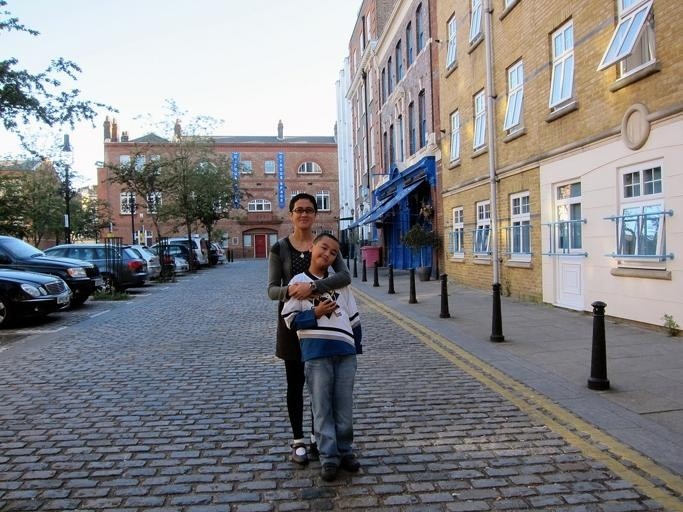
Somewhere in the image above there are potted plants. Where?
[405,221,441,281]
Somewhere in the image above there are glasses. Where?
[292,207,314,214]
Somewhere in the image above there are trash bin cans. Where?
[359,246,381,268]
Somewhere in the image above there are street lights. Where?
[59,133,73,244]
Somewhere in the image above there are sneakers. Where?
[341,454,360,472]
[320,462,337,482]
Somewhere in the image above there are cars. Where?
[0,234,225,328]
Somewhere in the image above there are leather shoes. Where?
[309,437,319,456]
[290,443,308,464]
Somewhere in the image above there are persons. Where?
[227,248,230,262]
[230,249,233,262]
[281,233,362,482]
[267,193,352,464]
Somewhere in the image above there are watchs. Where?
[309,282,317,294]
